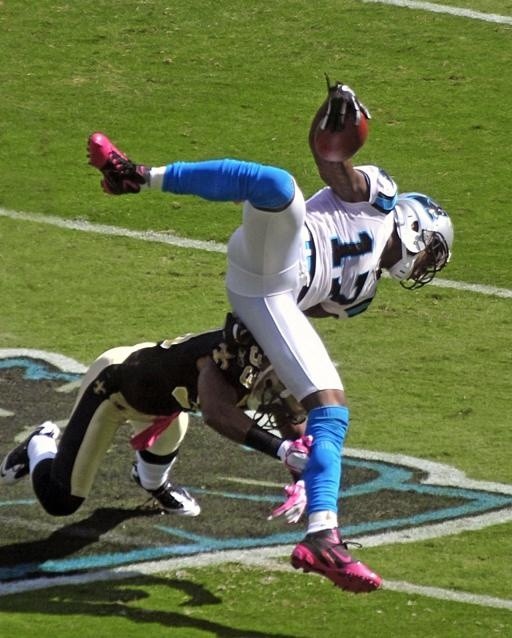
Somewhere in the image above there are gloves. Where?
[276,434,313,475]
[319,82,372,130]
[266,479,307,523]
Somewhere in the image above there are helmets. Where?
[388,192,454,283]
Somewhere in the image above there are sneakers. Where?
[0,420,63,486]
[290,526,382,594]
[86,131,147,194]
[129,462,201,518]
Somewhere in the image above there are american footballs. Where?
[313,110,368,162]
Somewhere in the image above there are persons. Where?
[88,85,455,594]
[0,313,312,527]
